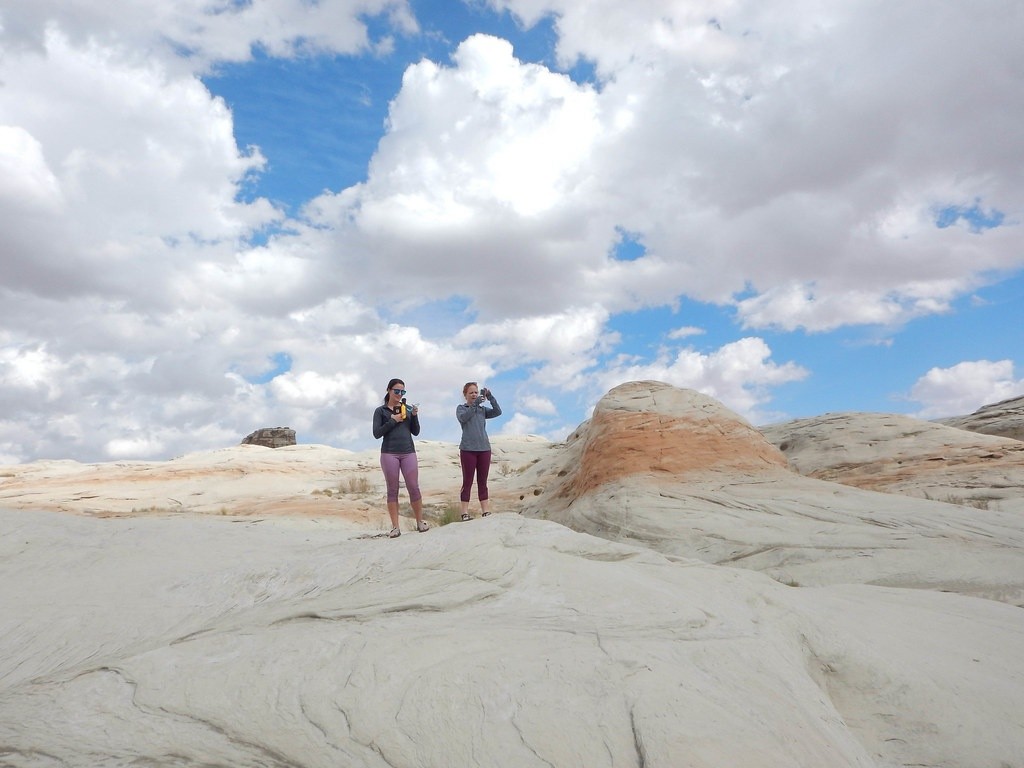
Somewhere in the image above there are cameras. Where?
[480,389,486,401]
[393,405,401,416]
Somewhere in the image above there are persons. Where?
[373,379,429,538]
[457,382,502,521]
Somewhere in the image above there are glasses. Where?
[390,389,406,395]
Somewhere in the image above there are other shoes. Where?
[461,513,470,520]
[482,512,491,518]
[417,522,429,532]
[390,529,401,537]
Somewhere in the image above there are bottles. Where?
[401,398,406,419]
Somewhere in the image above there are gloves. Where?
[480,388,490,396]
[475,396,485,405]
